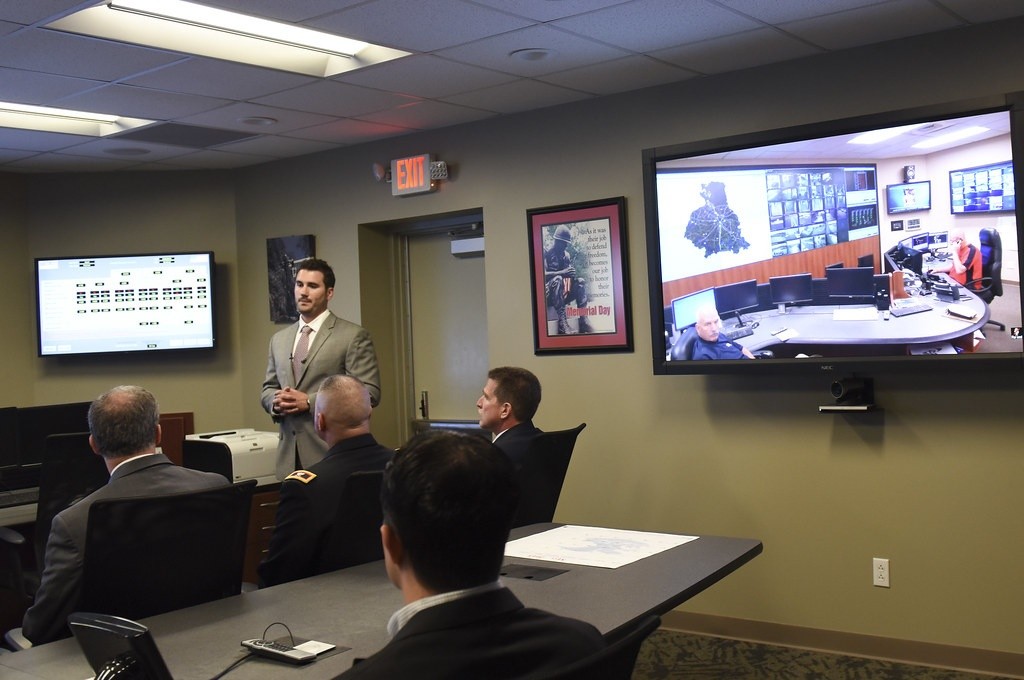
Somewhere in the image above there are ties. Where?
[291,326,314,385]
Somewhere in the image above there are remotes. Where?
[241,639,317,664]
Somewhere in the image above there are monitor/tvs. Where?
[34,251,218,357]
[640,90,1024,376]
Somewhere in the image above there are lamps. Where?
[372,154,447,197]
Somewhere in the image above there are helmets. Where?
[553,224,571,244]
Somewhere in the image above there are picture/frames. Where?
[526,195,633,356]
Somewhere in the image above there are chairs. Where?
[962,228,1006,337]
[670,327,696,360]
[305,473,384,576]
[5,480,257,652]
[499,423,586,523]
[0,432,109,609]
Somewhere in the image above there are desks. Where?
[1,524,766,680]
[666,271,990,362]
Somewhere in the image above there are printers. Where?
[182,428,280,482]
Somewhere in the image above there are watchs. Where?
[307,399,310,405]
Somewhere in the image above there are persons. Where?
[333,428,607,680]
[543,225,594,335]
[692,306,755,360]
[477,366,544,448]
[926,230,982,291]
[20,384,231,646]
[261,375,398,591]
[261,259,382,483]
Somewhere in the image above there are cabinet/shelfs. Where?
[244,492,282,584]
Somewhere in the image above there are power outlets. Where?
[872,559,889,588]
